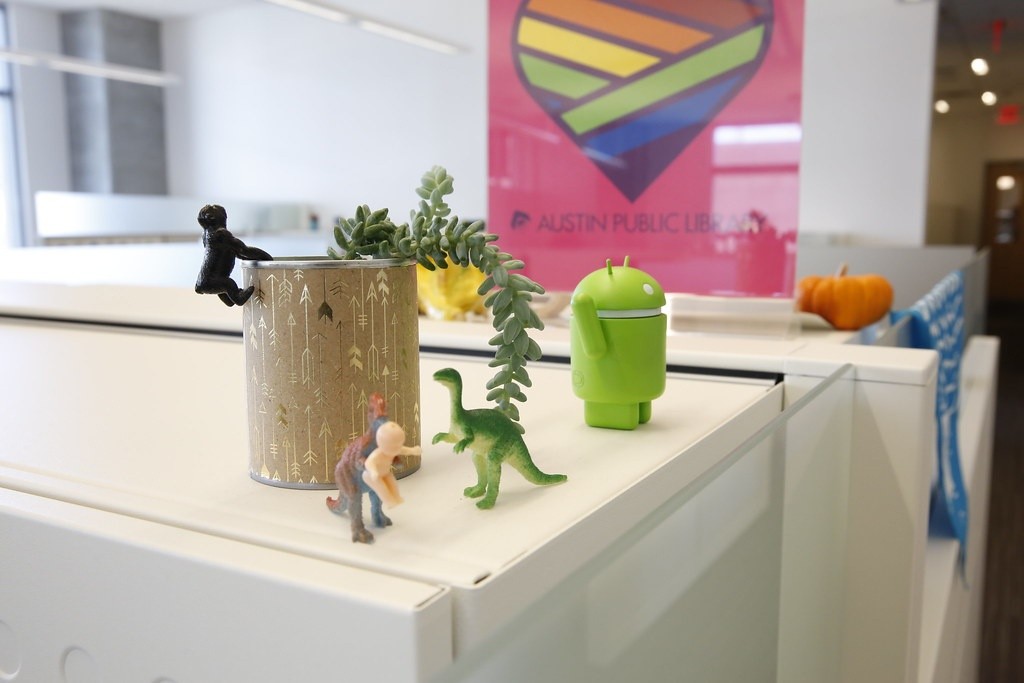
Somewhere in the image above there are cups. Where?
[240,256,423,492]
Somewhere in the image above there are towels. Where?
[889,269,972,593]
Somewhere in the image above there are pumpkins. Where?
[794,264,894,332]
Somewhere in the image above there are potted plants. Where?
[240,165,549,490]
